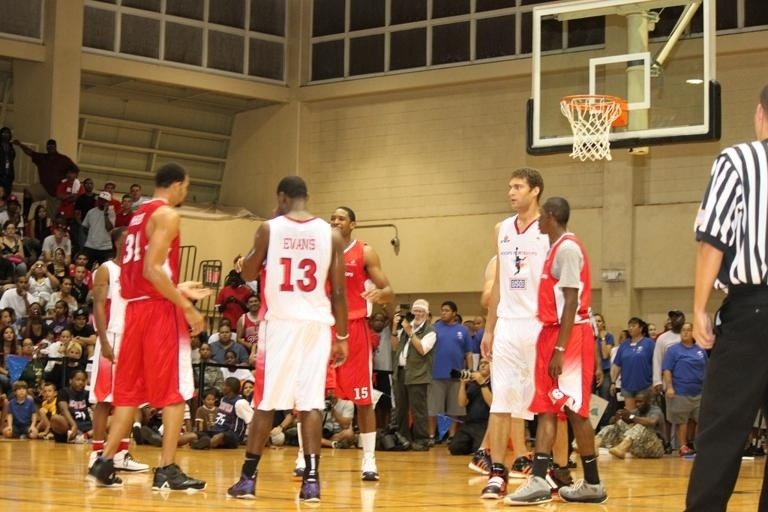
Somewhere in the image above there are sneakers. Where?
[189,436,210,450]
[293,455,307,478]
[427,435,455,449]
[84,457,124,488]
[151,462,207,493]
[358,455,380,480]
[502,474,555,506]
[678,444,689,457]
[226,468,259,500]
[330,439,350,449]
[111,447,150,473]
[509,451,538,479]
[609,445,626,460]
[140,424,164,448]
[556,483,609,506]
[467,447,493,476]
[298,475,320,503]
[480,463,510,500]
[544,465,574,492]
[87,448,102,468]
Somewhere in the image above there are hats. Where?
[98,191,113,202]
[412,298,430,312]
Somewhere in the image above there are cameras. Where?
[32,310,39,314]
[36,264,42,268]
[398,304,415,328]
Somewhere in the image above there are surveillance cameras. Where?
[391,238,399,246]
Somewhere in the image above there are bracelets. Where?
[409,332,413,338]
[601,338,606,340]
[335,333,349,340]
[480,384,488,387]
[555,346,565,351]
[104,213,108,216]
[610,382,614,385]
[629,414,634,420]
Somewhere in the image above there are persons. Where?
[227,175,350,504]
[2,191,96,443]
[683,83,768,512]
[88,229,149,471]
[294,207,394,481]
[56,168,143,270]
[12,139,80,224]
[588,310,685,458]
[1,129,16,201]
[269,396,364,449]
[468,222,549,477]
[661,323,711,458]
[549,308,603,468]
[504,197,608,505]
[480,168,568,500]
[133,253,261,451]
[86,163,214,491]
[373,298,492,456]
